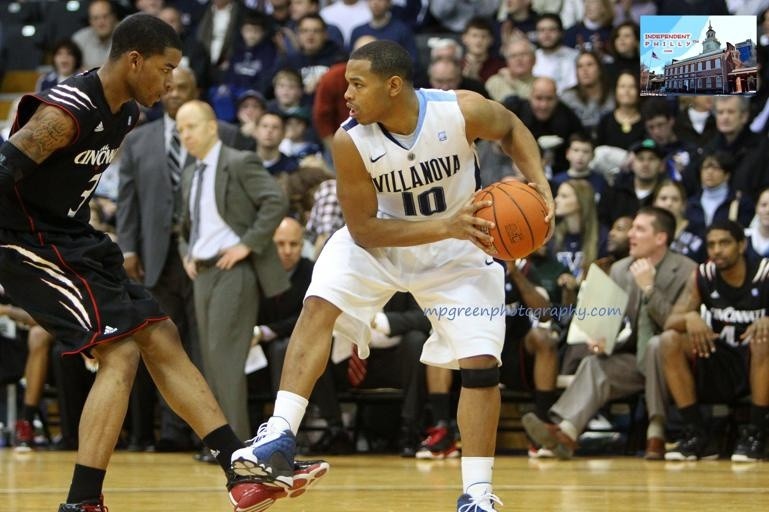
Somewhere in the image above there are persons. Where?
[484,39,539,103]
[532,11,581,96]
[560,50,617,138]
[191,0,261,84]
[309,292,432,458]
[347,1,417,63]
[461,18,501,80]
[268,0,295,37]
[72,2,115,72]
[614,0,655,26]
[281,113,321,163]
[278,14,350,103]
[417,260,558,460]
[742,190,769,259]
[51,341,131,452]
[312,34,380,171]
[644,109,694,151]
[431,39,463,61]
[612,21,640,71]
[660,219,769,462]
[175,99,289,466]
[522,206,698,461]
[1,13,331,512]
[652,178,709,265]
[677,95,725,157]
[703,96,769,190]
[685,149,742,229]
[272,68,321,144]
[119,65,245,453]
[610,139,666,222]
[245,215,316,425]
[33,39,83,95]
[287,166,345,260]
[428,57,491,101]
[210,10,277,121]
[596,71,649,150]
[137,1,164,15]
[501,78,591,166]
[579,216,634,285]
[2,288,54,454]
[158,8,212,90]
[566,1,616,71]
[230,41,556,512]
[530,179,609,305]
[500,0,545,47]
[265,71,321,144]
[282,106,320,160]
[549,134,610,195]
[318,0,374,48]
[273,0,344,71]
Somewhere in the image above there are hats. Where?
[630,138,668,157]
[235,89,267,108]
[280,106,309,122]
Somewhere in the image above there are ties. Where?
[187,163,207,263]
[638,290,655,378]
[162,127,181,227]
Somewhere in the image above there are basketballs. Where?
[471,178,548,262]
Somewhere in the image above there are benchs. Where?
[249,383,741,459]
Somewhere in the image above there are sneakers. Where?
[456,493,499,512]
[15,416,32,453]
[354,435,371,453]
[665,439,700,461]
[444,439,460,459]
[415,428,446,460]
[228,459,330,512]
[698,442,720,459]
[731,439,762,461]
[54,500,108,512]
[400,440,415,456]
[527,444,539,458]
[230,423,296,488]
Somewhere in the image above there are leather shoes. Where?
[523,412,579,460]
[645,436,663,461]
[191,446,216,463]
